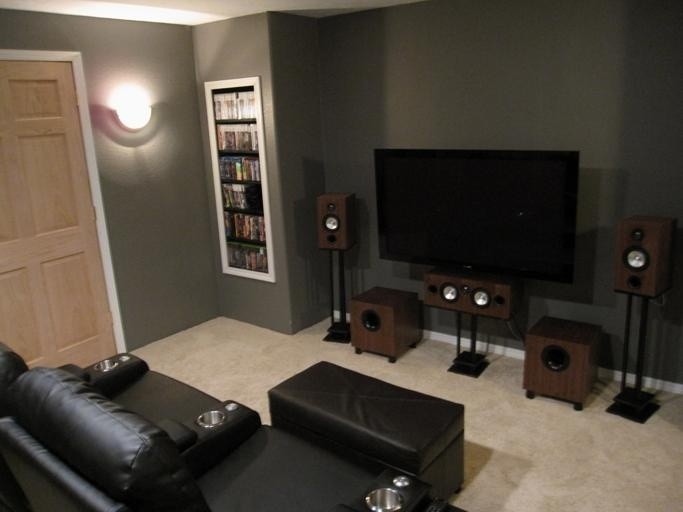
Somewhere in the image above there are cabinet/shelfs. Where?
[204,76,276,283]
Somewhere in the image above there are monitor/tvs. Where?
[372,146,580,286]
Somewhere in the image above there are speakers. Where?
[614,214,676,297]
[522,316,603,410]
[349,287,422,362]
[316,192,355,252]
[423,270,521,321]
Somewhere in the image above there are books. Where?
[208,91,269,274]
